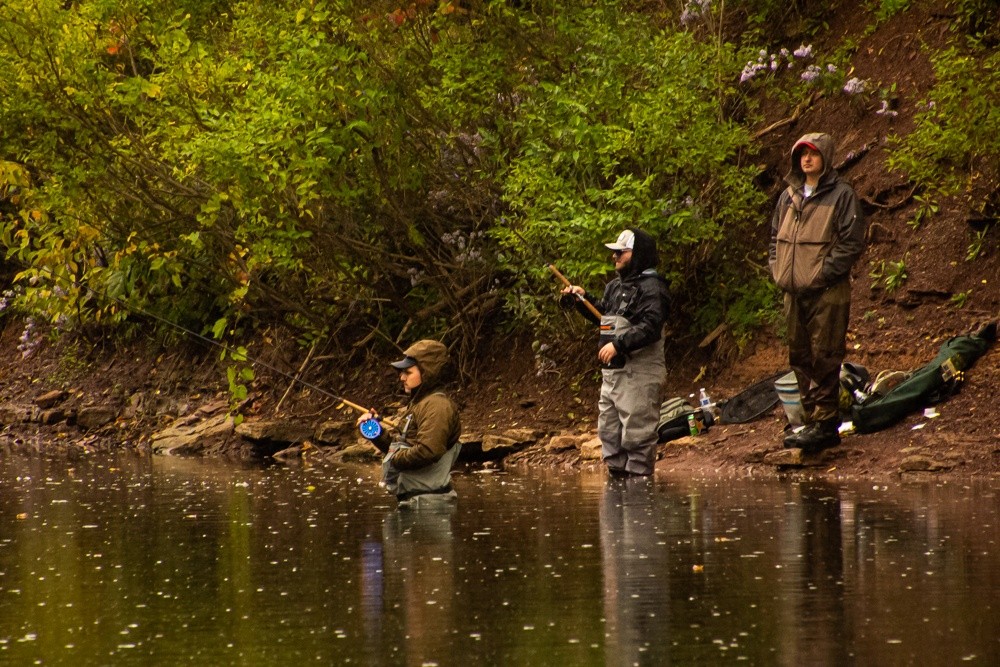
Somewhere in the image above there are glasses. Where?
[613,250,632,256]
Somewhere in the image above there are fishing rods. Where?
[488,205,634,361]
[0,249,404,440]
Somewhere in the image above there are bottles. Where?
[699,388,712,407]
[689,394,701,408]
[854,389,867,404]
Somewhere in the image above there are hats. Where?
[604,229,635,250]
[389,356,417,369]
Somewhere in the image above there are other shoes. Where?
[796,417,841,448]
[783,421,816,448]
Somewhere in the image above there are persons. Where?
[355,339,462,512]
[768,133,865,451]
[560,227,670,479]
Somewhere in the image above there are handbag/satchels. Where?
[656,396,697,441]
